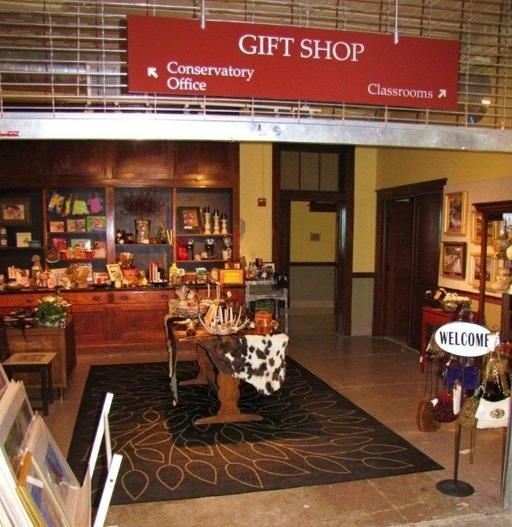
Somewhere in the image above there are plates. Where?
[151,282,168,288]
[90,282,113,289]
[150,240,164,243]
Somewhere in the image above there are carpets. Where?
[63,352,446,511]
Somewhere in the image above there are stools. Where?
[2,350,59,418]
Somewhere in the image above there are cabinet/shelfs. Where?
[471,196,512,375]
[0,183,241,281]
[0,138,239,187]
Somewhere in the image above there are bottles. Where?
[116,228,132,244]
[169,262,219,285]
[187,237,232,260]
[491,216,512,259]
[245,257,288,291]
[224,257,240,269]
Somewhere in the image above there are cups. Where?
[0,226,9,246]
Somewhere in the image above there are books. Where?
[0,361,90,527]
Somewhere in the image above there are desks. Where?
[166,305,294,428]
[0,284,250,356]
[2,310,79,386]
[245,278,290,334]
[418,302,478,374]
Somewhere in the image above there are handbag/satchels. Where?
[451,383,463,416]
[434,389,458,423]
[475,397,512,430]
[458,397,482,429]
[444,358,482,390]
[416,398,440,432]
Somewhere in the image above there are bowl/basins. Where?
[29,240,42,247]
[424,298,442,308]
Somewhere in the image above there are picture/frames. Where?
[468,251,496,289]
[469,207,497,245]
[441,240,466,279]
[442,187,469,236]
